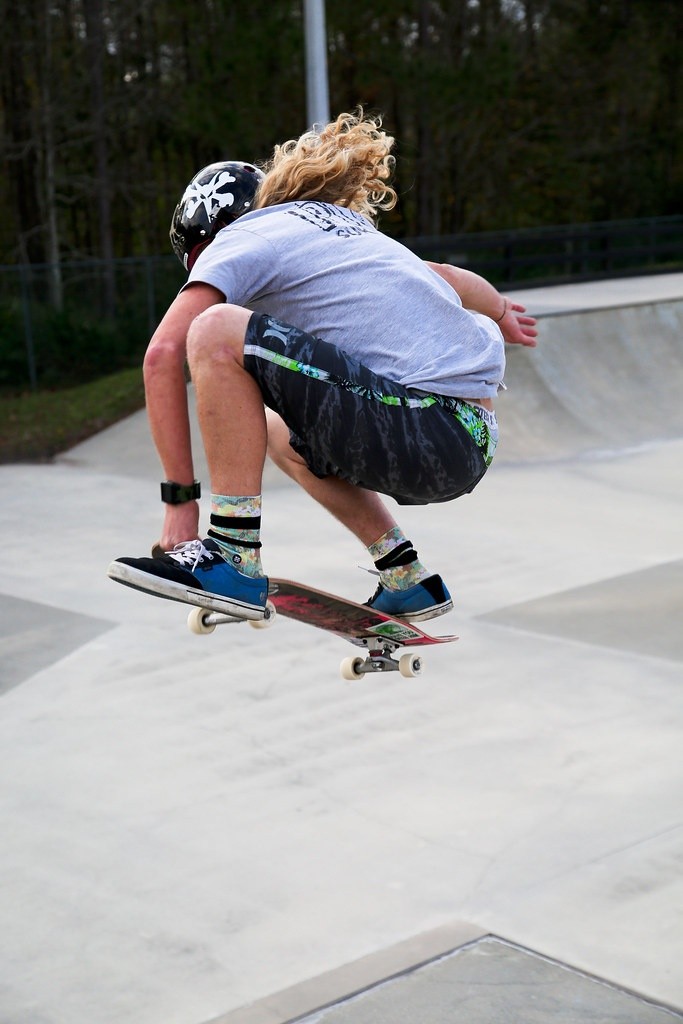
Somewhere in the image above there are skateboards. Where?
[190,572,459,679]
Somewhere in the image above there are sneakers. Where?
[357,566,454,623]
[109,540,269,621]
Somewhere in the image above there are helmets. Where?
[169,161,266,273]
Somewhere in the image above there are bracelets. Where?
[497,298,506,322]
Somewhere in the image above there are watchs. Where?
[160,479,201,505]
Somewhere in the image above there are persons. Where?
[108,113,539,624]
